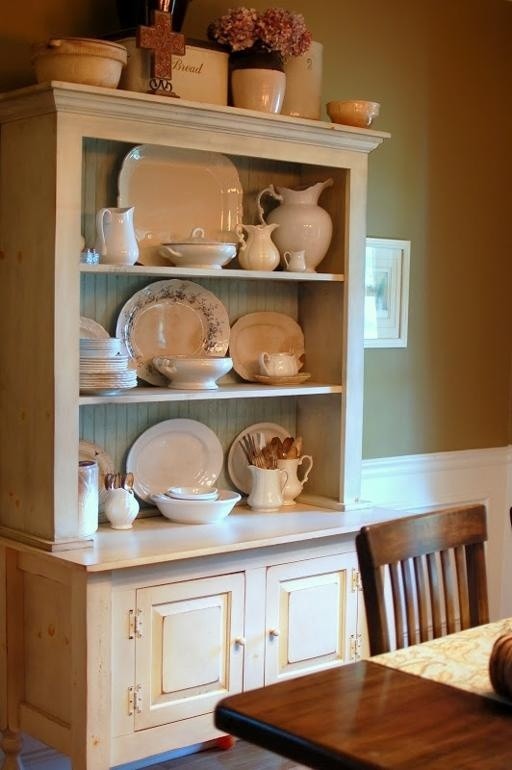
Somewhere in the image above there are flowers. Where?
[211,5,314,68]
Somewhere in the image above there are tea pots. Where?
[234,221,280,271]
[246,465,289,512]
[283,248,307,272]
[94,204,141,267]
[258,351,305,377]
[253,175,337,273]
[278,456,314,505]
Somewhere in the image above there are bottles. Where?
[81,248,92,263]
[89,248,101,265]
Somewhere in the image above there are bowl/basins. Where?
[149,353,234,390]
[325,97,382,128]
[27,35,130,89]
[154,244,239,269]
[149,489,242,526]
[163,484,221,503]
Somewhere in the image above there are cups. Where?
[78,460,100,539]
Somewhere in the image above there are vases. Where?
[231,65,287,117]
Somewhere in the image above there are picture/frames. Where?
[365,237,411,348]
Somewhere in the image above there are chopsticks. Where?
[236,431,258,466]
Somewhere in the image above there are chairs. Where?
[356,503,491,657]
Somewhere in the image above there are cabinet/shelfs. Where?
[1,81,391,769]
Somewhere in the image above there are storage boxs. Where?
[114,37,231,106]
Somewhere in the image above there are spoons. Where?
[102,471,138,494]
[262,433,301,469]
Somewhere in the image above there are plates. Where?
[79,439,117,525]
[228,311,308,380]
[253,373,313,387]
[79,338,139,397]
[114,144,245,267]
[227,421,294,496]
[115,279,231,387]
[125,417,224,506]
[79,316,111,338]
